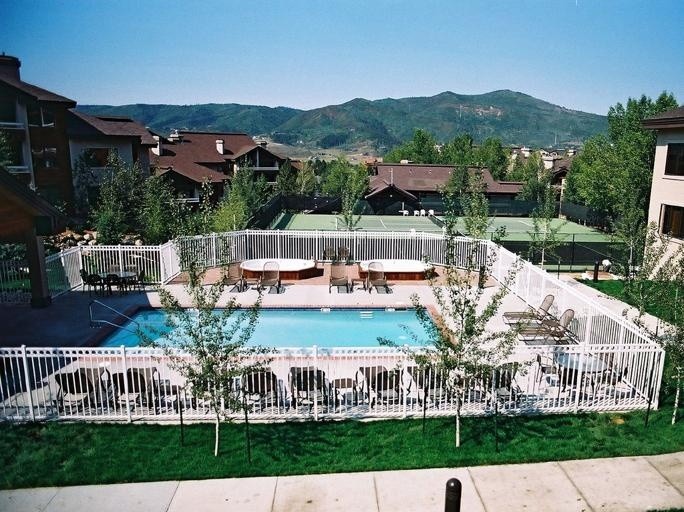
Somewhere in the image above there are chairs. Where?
[502,296,575,344]
[329,263,349,293]
[219,259,246,292]
[367,261,388,294]
[0,341,627,417]
[257,262,282,294]
[79,268,145,297]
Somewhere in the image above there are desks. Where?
[351,278,366,292]
[243,277,258,291]
